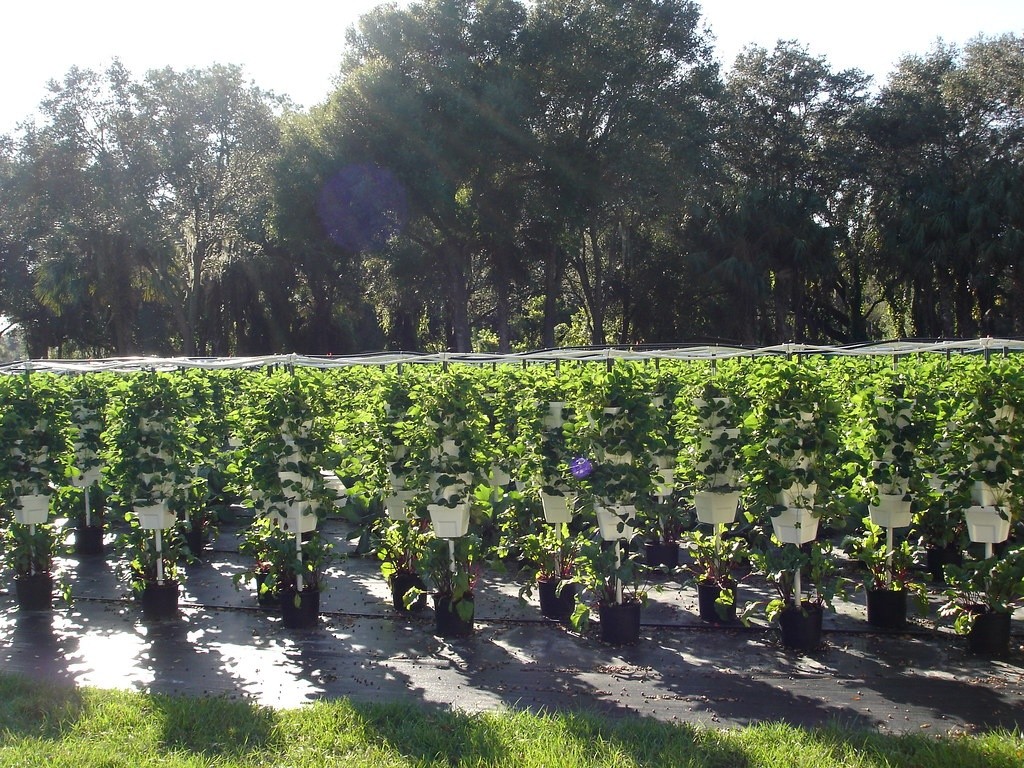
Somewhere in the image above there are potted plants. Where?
[0,348,1024,652]
